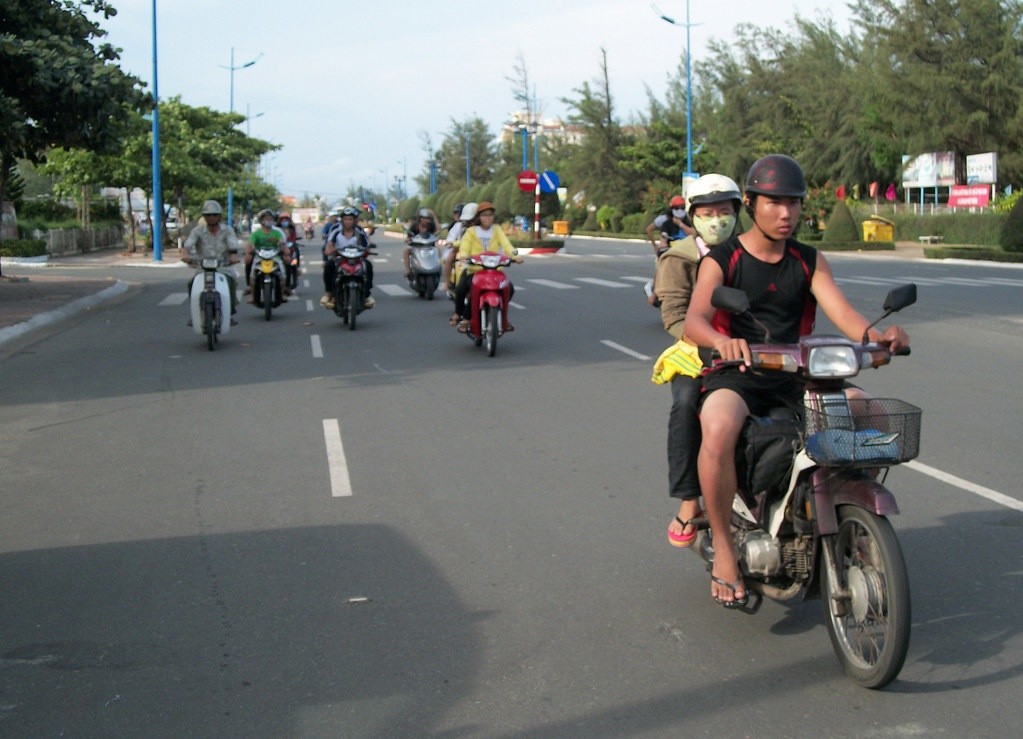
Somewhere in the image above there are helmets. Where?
[202,200,222,214]
[418,209,433,223]
[669,196,685,208]
[746,155,808,197]
[477,201,495,216]
[340,207,355,218]
[686,174,744,219]
[258,209,274,220]
[278,212,290,221]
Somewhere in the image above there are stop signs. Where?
[518,171,538,193]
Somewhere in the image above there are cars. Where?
[137,216,179,235]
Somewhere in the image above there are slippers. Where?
[668,510,702,548]
[711,571,748,610]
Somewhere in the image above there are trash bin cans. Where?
[552,219,569,235]
[861,213,896,243]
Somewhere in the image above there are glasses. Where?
[205,214,217,217]
[673,207,685,211]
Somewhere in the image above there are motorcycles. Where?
[643,231,688,307]
[692,285,926,687]
[301,223,314,240]
[181,249,242,350]
[401,222,449,301]
[451,250,519,357]
[326,242,378,330]
[250,237,305,320]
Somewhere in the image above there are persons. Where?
[179,199,240,327]
[646,195,697,303]
[243,201,520,332]
[685,154,910,609]
[655,172,744,548]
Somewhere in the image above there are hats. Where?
[460,203,479,221]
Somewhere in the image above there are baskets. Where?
[796,398,923,467]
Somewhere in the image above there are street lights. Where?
[662,16,695,174]
[227,61,263,230]
[511,124,536,232]
[369,161,413,226]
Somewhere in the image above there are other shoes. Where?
[319,296,330,306]
[326,298,335,309]
[187,319,192,326]
[457,320,470,333]
[365,295,376,307]
[505,322,514,332]
[449,315,460,328]
[230,318,238,326]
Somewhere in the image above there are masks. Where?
[673,210,685,217]
[692,215,735,244]
[263,220,274,227]
[281,222,289,227]
